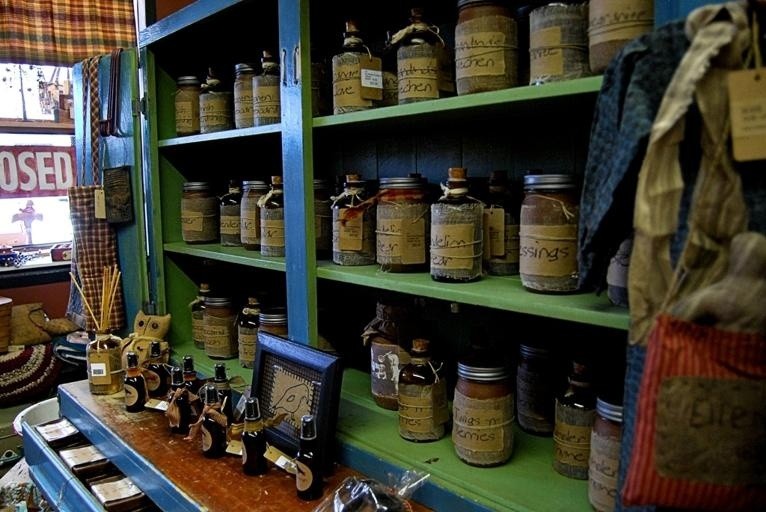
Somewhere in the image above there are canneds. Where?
[242,179,267,248]
[205,319,208,321]
[455,0,518,95]
[180,181,218,245]
[254,307,288,338]
[204,295,237,360]
[377,176,431,272]
[313,179,332,260]
[453,359,516,467]
[518,173,579,293]
[517,342,558,437]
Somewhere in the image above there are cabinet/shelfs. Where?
[21,1,631,512]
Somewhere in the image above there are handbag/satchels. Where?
[621,313,766,510]
[12,303,51,346]
[44,318,80,334]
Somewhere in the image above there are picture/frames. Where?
[250,330,344,476]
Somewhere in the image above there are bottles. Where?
[314,165,579,295]
[370,300,622,512]
[192,282,287,369]
[181,174,285,257]
[174,60,281,137]
[295,415,322,501]
[309,0,654,117]
[86,329,269,477]
